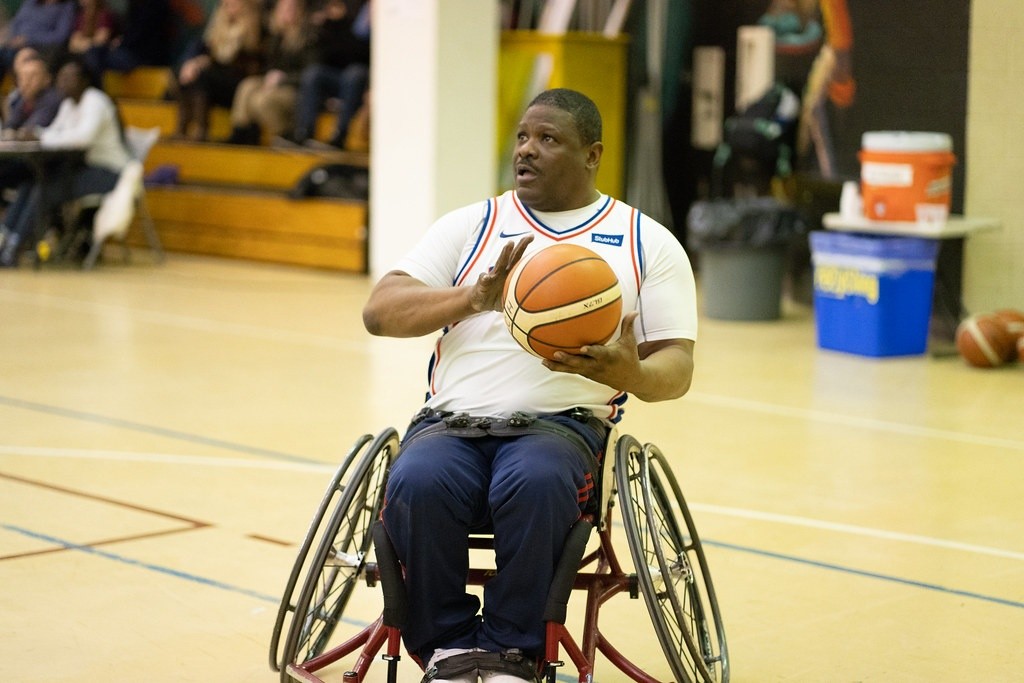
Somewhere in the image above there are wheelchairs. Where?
[268,427,731,683]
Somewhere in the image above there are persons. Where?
[361,87,699,683]
[0,0,372,275]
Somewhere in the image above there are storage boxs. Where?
[810,230,940,356]
[857,149,956,221]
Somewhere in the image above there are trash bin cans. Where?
[691,198,791,320]
[809,228,941,357]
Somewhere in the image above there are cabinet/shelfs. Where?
[497,29,627,202]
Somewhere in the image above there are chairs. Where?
[63,125,163,268]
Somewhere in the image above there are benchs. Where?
[104,68,370,268]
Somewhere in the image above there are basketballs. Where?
[957,314,1010,368]
[1000,309,1024,362]
[501,241,622,361]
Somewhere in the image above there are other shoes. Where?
[475,647,536,682]
[426,648,476,683]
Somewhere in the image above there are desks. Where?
[0,141,45,154]
